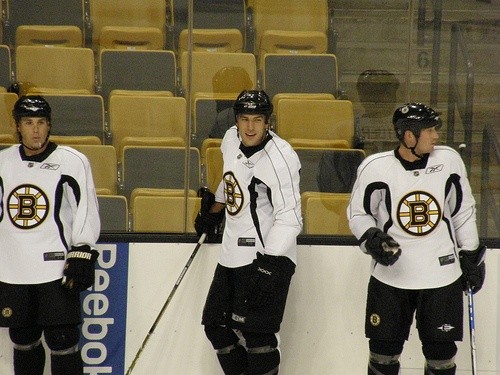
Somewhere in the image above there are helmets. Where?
[12,94,52,121]
[392,102,442,139]
[232,89,274,117]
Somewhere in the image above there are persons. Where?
[347,102,484,375]
[194,89,302,375]
[320,70,409,239]
[0,93,101,375]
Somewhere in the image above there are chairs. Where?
[0,0,362,241]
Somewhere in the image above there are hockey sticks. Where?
[466,280,477,375]
[126,232,207,375]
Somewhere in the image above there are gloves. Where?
[60,244,100,295]
[458,243,487,296]
[356,226,403,268]
[241,251,297,306]
[193,187,225,243]
